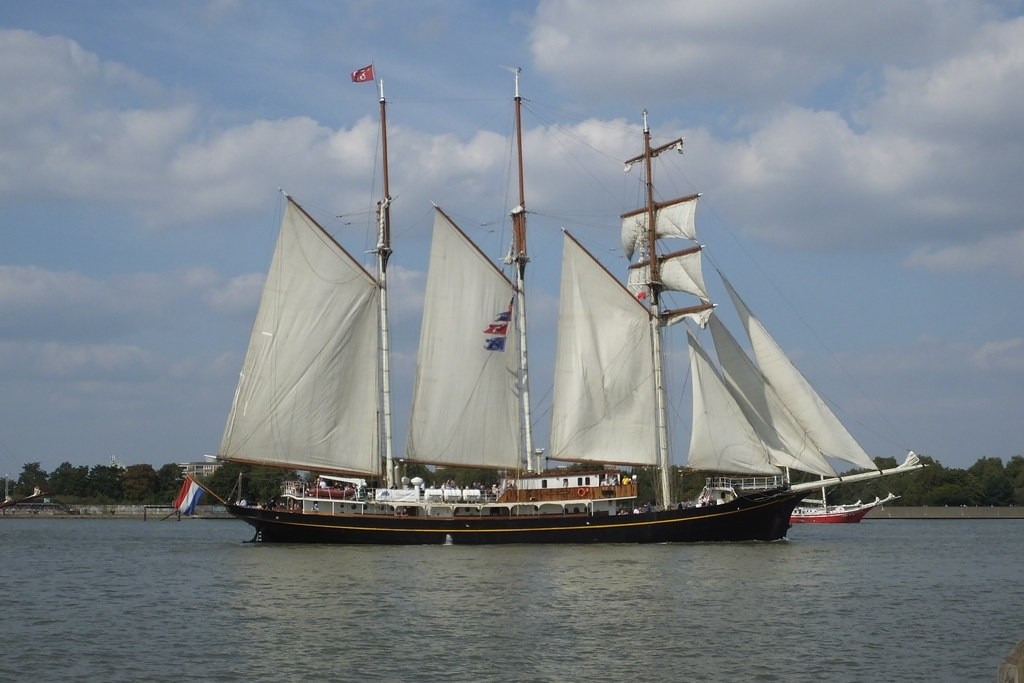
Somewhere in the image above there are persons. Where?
[701,496,717,507]
[616,509,621,515]
[234,498,247,507]
[634,508,639,514]
[257,500,272,511]
[441,479,485,489]
[295,504,303,513]
[391,483,398,490]
[601,475,631,487]
[677,502,682,510]
[319,478,351,490]
[492,481,512,493]
[311,504,320,512]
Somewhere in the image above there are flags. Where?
[485,337,506,352]
[351,64,373,82]
[485,324,508,335]
[174,475,204,515]
[496,311,511,321]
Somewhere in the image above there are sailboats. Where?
[789,473,901,525]
[216,68,813,546]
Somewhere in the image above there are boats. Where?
[305,486,355,499]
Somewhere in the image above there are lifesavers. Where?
[577,488,586,498]
